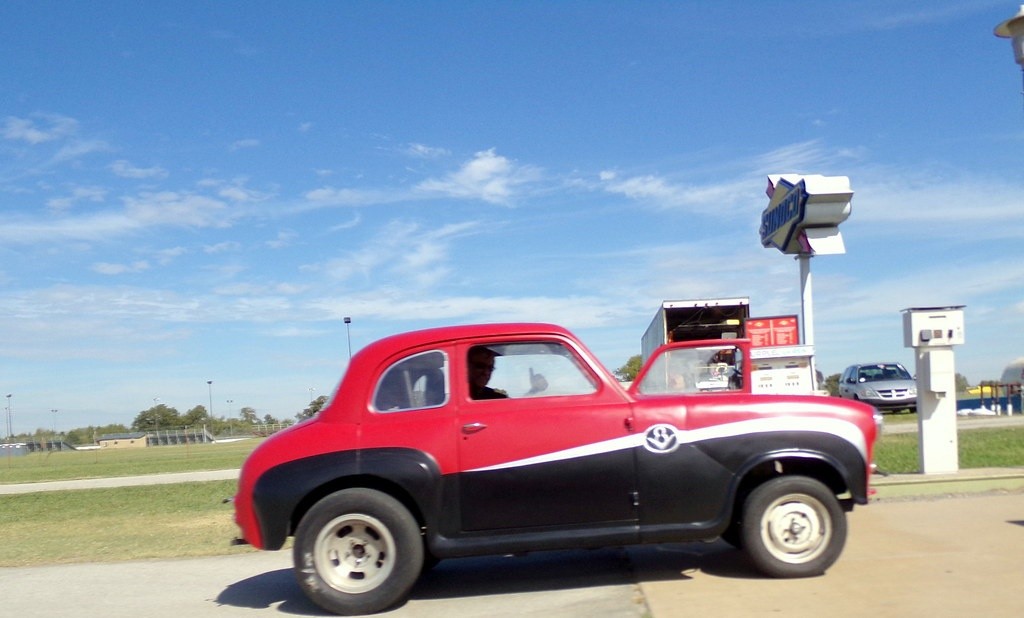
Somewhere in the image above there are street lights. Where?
[232,321,885,618]
[227,399,233,417]
[5,407,9,436]
[51,409,58,432]
[344,317,352,359]
[7,393,12,437]
[207,380,214,436]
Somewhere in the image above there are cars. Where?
[840,364,919,414]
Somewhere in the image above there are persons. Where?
[468,345,549,400]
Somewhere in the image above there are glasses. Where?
[473,362,495,372]
